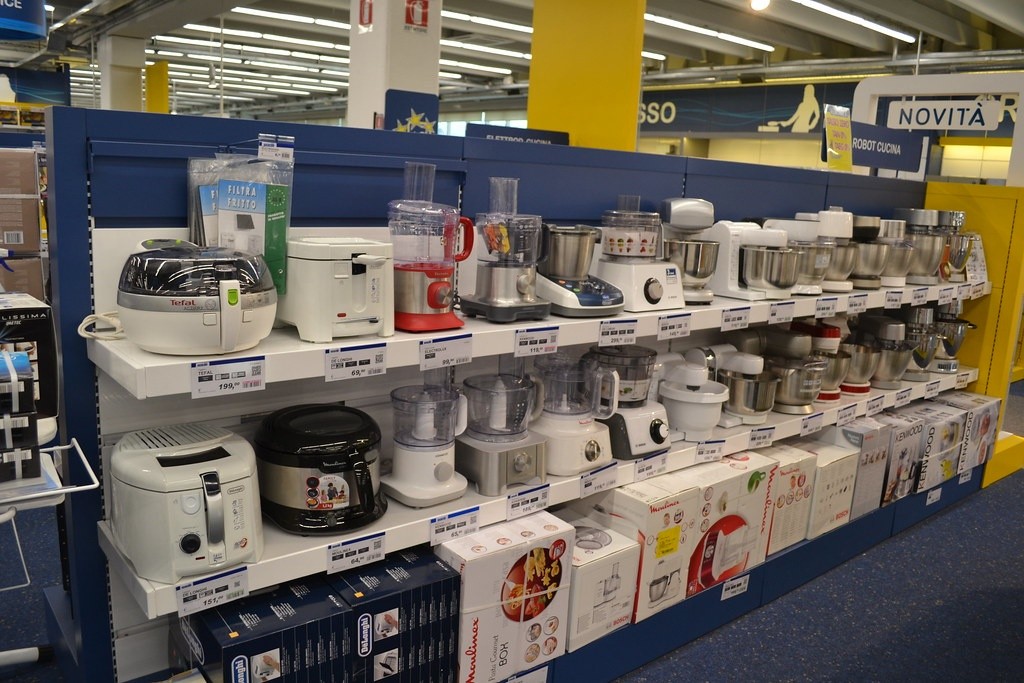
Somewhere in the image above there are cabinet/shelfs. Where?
[43,105,988,683]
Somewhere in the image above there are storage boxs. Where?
[563,472,700,624]
[549,507,641,653]
[668,450,780,601]
[168,573,355,683]
[316,546,461,683]
[778,435,860,543]
[749,440,818,556]
[433,509,576,683]
[0,148,59,484]
[825,388,1002,521]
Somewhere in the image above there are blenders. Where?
[111,156,978,588]
[252,515,761,682]
